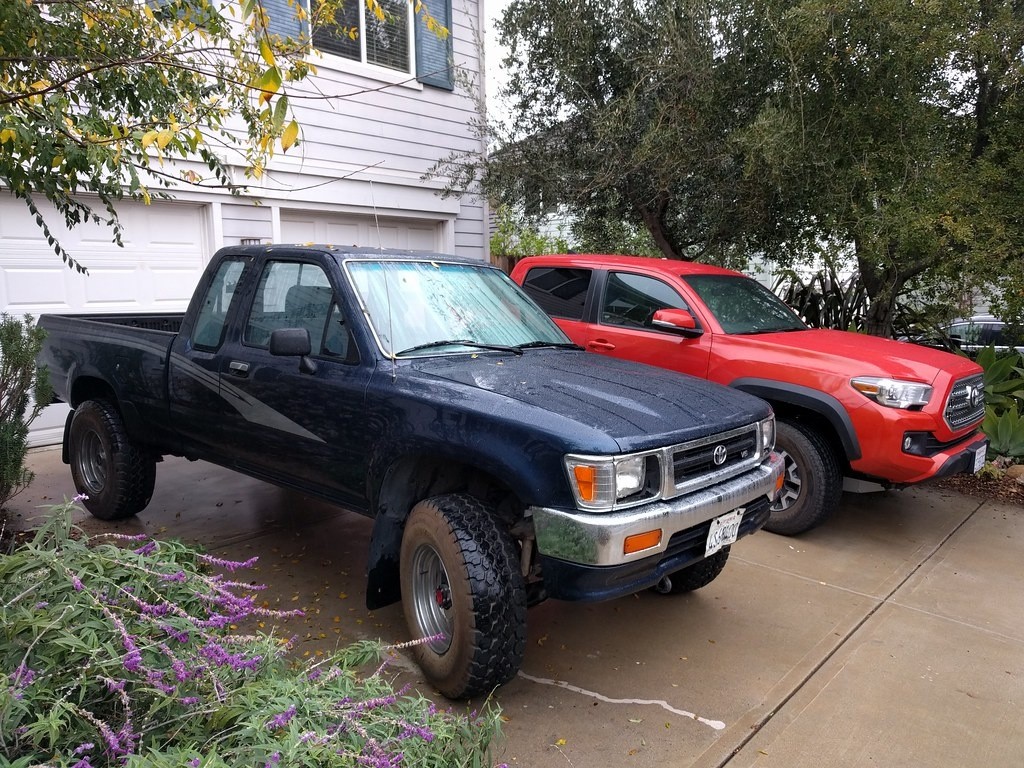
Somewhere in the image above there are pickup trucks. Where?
[508,254,994,535]
[34,241,785,706]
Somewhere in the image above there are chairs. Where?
[285,285,336,318]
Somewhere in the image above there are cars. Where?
[896,316,1024,355]
